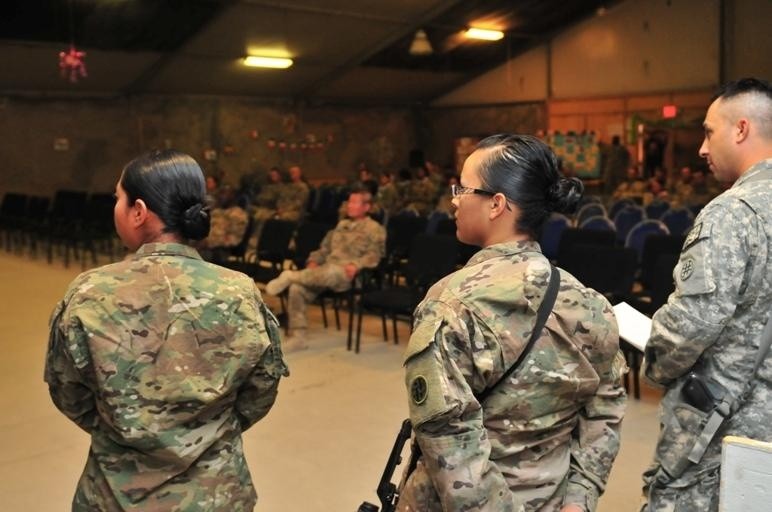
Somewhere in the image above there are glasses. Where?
[451,185,512,212]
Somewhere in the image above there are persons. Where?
[639,77,772,512]
[201,159,458,355]
[597,129,734,210]
[43,147,290,512]
[394,132,630,511]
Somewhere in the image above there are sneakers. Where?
[265,269,292,296]
[280,328,308,353]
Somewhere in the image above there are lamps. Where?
[409,29,433,55]
